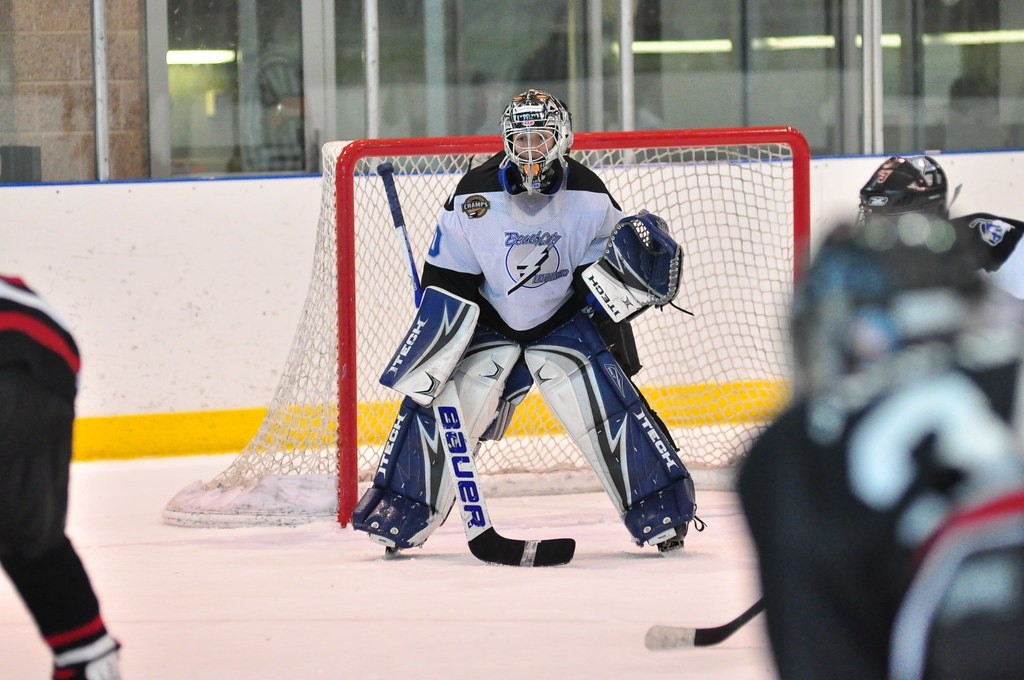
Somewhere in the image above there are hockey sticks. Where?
[376,160,575,566]
[644,597,766,651]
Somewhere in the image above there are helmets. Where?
[860,154,947,226]
[500,89,572,189]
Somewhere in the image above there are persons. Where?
[348,88,696,559]
[0,274,122,680]
[736,150,1024,680]
